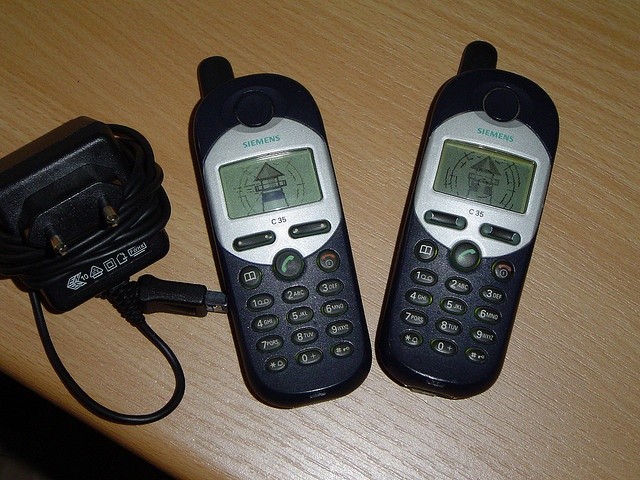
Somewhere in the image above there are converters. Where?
[1,115,171,315]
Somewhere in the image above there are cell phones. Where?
[188,55,374,409]
[375,42,560,399]
